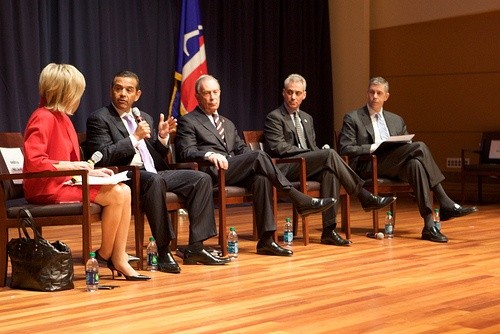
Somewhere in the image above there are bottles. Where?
[85,252,99,294]
[433,209,441,232]
[227,227,239,258]
[384,211,393,240]
[283,218,294,248]
[146,237,158,271]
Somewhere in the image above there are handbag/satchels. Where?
[8,208,75,292]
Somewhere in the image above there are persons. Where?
[176,74,337,255]
[264,74,397,245]
[22,62,150,279]
[86,70,231,273]
[338,76,478,242]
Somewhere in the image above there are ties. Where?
[293,111,309,149]
[375,114,389,140]
[122,113,157,174]
[212,114,225,142]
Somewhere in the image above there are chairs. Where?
[76,132,198,270]
[461,130,500,202]
[0,132,119,288]
[243,130,351,245]
[167,138,277,257]
[333,129,434,236]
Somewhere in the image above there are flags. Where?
[168,0,209,135]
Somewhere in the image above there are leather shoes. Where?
[440,204,475,221]
[320,231,352,245]
[422,226,448,242]
[182,248,231,265]
[157,252,181,273]
[257,241,293,256]
[295,197,337,217]
[361,196,397,212]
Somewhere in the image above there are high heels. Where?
[107,257,151,281]
[95,249,140,265]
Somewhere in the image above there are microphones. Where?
[71,151,103,183]
[132,107,142,122]
[367,232,384,239]
[322,144,329,150]
